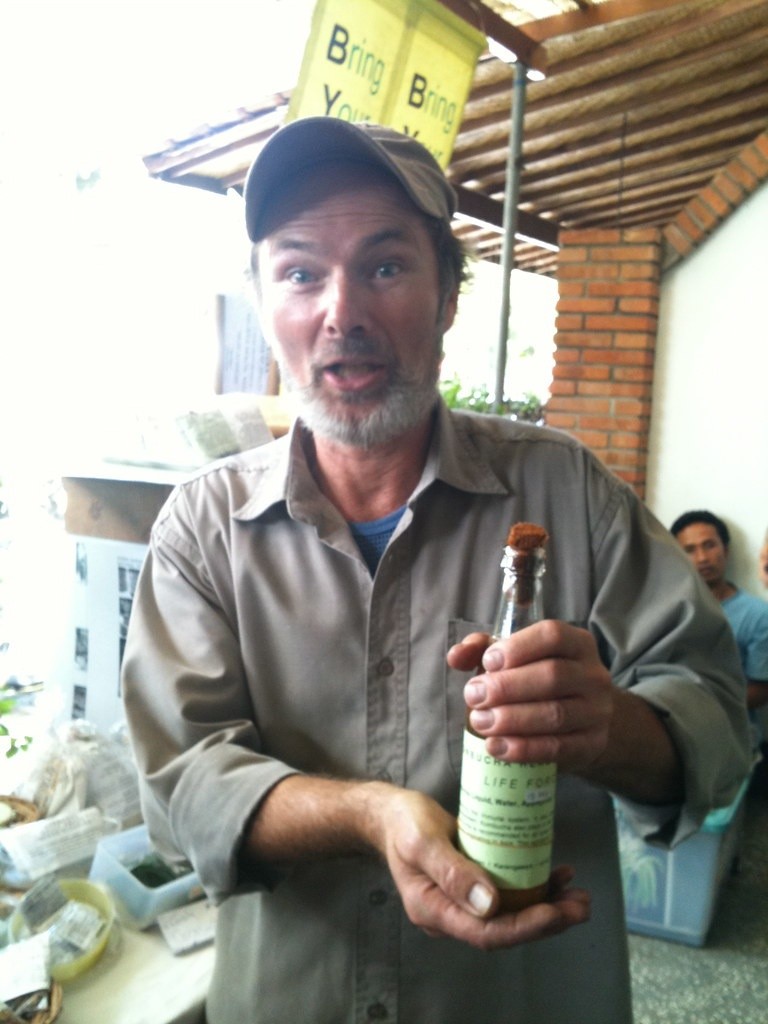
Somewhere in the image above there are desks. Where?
[0,858,211,1024]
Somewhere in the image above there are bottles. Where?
[455,523,557,909]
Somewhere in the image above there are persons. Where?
[671,512,768,707]
[119,115,768,1024]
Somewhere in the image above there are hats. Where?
[243,117,458,242]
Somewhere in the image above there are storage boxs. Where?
[609,756,760,948]
[85,822,207,927]
